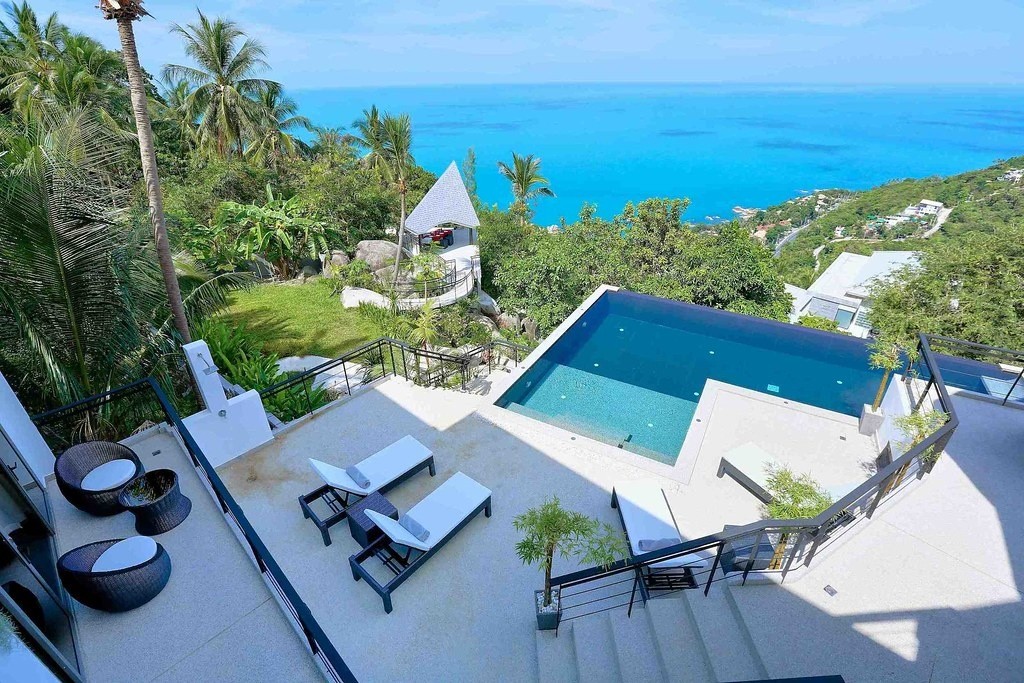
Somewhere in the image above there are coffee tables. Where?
[119,468,192,536]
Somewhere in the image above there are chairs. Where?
[57,536,171,613]
[612,484,707,607]
[350,471,492,611]
[298,434,435,546]
[54,441,146,516]
[716,440,855,544]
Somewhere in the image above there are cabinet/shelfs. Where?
[719,524,773,576]
[346,490,398,548]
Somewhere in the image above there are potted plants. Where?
[514,496,633,630]
[858,321,919,435]
